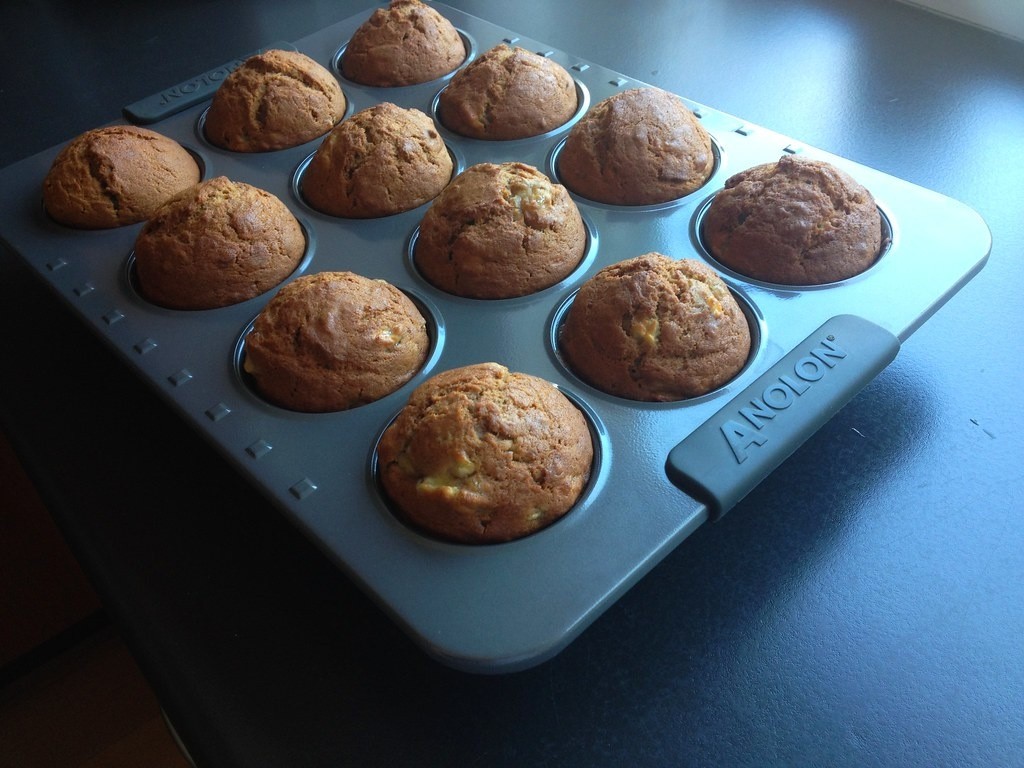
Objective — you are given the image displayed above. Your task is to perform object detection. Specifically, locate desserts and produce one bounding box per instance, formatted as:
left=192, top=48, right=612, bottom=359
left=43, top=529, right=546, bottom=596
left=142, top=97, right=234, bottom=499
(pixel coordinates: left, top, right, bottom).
left=43, top=0, right=880, bottom=541
